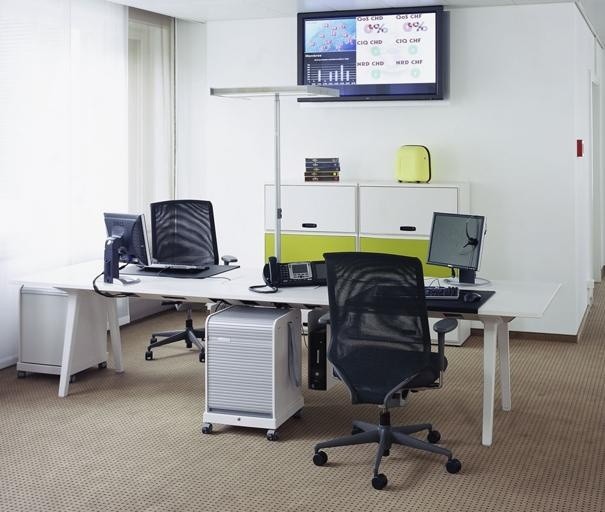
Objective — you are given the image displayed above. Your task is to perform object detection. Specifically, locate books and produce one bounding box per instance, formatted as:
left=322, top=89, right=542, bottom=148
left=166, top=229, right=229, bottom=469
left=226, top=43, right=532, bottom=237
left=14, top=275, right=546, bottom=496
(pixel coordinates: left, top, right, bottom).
left=305, top=158, right=340, bottom=182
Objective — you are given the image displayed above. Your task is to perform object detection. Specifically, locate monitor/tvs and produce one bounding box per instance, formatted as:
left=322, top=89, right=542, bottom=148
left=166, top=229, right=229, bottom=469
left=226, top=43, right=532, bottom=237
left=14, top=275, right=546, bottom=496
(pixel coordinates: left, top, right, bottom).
left=297, top=5, right=443, bottom=102
left=426, top=211, right=486, bottom=284
left=103, top=213, right=152, bottom=283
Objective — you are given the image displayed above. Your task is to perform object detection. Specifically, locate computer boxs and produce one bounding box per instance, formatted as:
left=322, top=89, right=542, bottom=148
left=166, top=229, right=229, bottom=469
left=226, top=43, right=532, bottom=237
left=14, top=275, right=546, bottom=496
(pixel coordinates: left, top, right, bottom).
left=307, top=309, right=341, bottom=391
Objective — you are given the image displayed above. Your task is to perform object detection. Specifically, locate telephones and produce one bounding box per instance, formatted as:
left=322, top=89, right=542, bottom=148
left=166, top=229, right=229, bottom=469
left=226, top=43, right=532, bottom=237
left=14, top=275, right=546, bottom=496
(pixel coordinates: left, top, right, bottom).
left=249, top=257, right=313, bottom=293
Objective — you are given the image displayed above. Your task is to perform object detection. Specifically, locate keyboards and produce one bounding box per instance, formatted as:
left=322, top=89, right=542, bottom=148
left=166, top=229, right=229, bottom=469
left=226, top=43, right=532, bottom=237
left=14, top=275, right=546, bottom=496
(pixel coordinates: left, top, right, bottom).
left=139, top=264, right=211, bottom=274
left=425, top=286, right=460, bottom=300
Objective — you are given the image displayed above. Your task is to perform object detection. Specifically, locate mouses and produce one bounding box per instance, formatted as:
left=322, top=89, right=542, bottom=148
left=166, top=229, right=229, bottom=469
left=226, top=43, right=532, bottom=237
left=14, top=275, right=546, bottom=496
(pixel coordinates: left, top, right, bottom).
left=462, top=292, right=482, bottom=304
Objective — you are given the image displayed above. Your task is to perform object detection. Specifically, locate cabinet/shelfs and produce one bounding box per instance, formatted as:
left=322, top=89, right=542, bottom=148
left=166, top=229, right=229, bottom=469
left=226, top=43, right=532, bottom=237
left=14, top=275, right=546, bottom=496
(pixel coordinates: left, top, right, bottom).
left=264, top=184, right=472, bottom=346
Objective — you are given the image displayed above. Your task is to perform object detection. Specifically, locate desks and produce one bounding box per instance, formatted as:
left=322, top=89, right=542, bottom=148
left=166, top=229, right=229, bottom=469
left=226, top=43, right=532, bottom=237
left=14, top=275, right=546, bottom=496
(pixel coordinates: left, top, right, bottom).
left=10, top=256, right=564, bottom=446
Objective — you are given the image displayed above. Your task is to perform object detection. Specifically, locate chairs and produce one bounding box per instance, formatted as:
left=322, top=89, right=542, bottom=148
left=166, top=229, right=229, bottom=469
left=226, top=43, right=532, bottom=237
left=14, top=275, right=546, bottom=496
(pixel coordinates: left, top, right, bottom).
left=313, top=252, right=461, bottom=490
left=144, top=199, right=238, bottom=363
